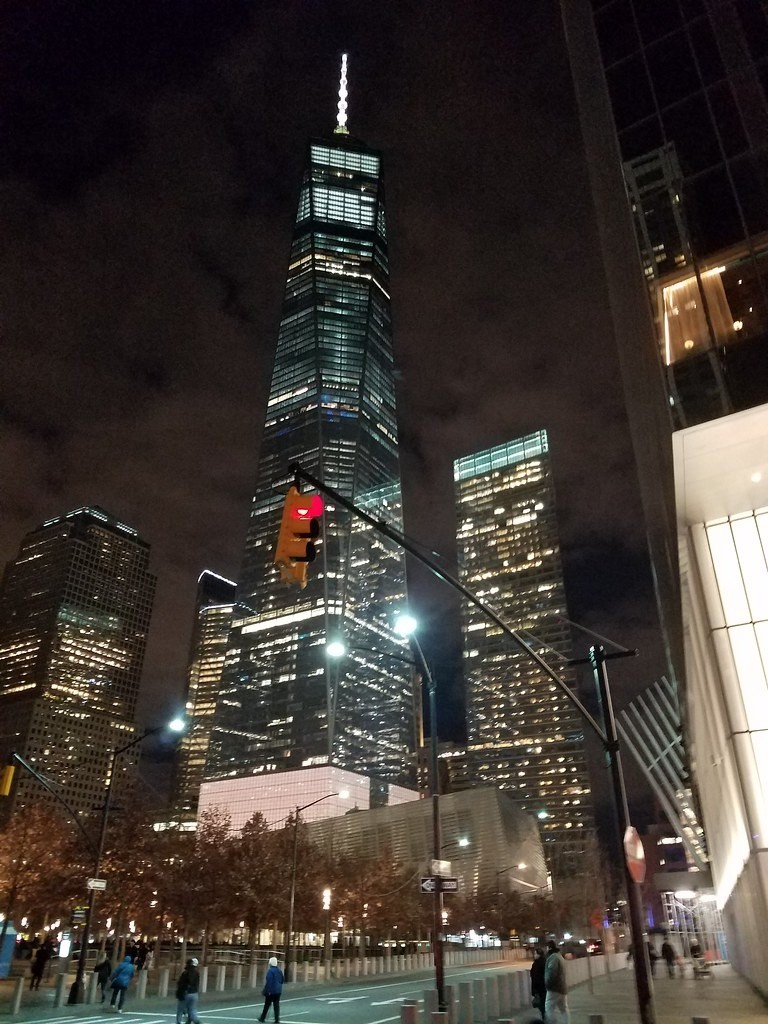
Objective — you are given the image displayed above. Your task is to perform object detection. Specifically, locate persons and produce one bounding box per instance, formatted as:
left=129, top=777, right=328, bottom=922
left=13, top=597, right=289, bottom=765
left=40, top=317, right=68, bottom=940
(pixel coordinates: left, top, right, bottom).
left=94, top=956, right=111, bottom=1004
left=44, top=936, right=56, bottom=956
left=140, top=943, right=155, bottom=969
left=176, top=958, right=199, bottom=1024
left=91, top=937, right=124, bottom=959
left=626, top=937, right=704, bottom=980
left=30, top=944, right=48, bottom=991
left=125, top=938, right=138, bottom=963
left=109, top=955, right=134, bottom=1014
left=525, top=938, right=572, bottom=1023
left=24, top=934, right=42, bottom=960
left=257, top=956, right=284, bottom=1024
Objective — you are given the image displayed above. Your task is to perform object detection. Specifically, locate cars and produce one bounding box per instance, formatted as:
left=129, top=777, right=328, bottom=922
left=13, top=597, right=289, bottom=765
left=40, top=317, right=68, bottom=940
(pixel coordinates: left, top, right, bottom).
left=585, top=939, right=604, bottom=956
left=558, top=941, right=589, bottom=960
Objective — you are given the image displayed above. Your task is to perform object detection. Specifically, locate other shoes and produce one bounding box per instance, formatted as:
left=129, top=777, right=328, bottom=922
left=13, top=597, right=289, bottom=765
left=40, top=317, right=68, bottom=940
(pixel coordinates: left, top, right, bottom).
left=118, top=1010, right=122, bottom=1013
left=257, top=1018, right=265, bottom=1023
left=110, top=1005, right=116, bottom=1009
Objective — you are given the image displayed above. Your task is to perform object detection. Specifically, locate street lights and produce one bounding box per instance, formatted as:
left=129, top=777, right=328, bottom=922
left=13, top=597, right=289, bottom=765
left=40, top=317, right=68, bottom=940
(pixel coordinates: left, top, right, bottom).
left=65, top=717, right=188, bottom=1007
left=396, top=611, right=448, bottom=1012
left=495, top=864, right=524, bottom=949
left=282, top=793, right=338, bottom=982
left=428, top=839, right=469, bottom=952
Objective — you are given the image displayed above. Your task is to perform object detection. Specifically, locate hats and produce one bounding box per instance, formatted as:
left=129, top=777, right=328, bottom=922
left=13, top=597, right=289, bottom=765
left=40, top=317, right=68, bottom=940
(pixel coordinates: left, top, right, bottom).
left=270, top=957, right=277, bottom=967
left=192, top=958, right=199, bottom=966
left=125, top=956, right=131, bottom=962
left=147, top=943, right=152, bottom=948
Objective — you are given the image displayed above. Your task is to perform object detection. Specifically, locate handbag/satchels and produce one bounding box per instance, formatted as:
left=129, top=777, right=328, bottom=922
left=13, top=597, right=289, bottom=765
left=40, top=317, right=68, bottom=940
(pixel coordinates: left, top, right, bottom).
left=110, top=977, right=119, bottom=989
